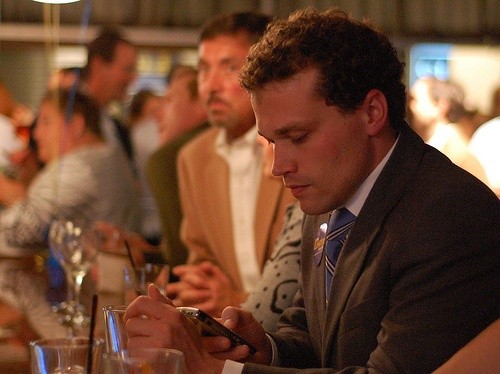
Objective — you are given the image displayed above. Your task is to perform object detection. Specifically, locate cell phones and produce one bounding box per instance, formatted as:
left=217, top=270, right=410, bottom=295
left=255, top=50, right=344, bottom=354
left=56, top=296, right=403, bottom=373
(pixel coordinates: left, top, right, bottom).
left=176, top=307, right=256, bottom=355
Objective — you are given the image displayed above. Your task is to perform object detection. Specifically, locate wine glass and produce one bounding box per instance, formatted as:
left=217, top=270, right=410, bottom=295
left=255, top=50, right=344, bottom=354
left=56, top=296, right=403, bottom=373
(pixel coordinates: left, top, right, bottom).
left=49, top=220, right=101, bottom=324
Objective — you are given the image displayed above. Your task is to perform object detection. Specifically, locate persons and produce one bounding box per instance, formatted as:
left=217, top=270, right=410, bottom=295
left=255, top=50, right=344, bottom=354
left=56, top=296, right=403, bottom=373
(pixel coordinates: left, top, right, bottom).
left=167, top=11, right=298, bottom=319
left=430, top=321, right=499, bottom=373
left=121, top=8, right=500, bottom=373
left=0, top=29, right=500, bottom=359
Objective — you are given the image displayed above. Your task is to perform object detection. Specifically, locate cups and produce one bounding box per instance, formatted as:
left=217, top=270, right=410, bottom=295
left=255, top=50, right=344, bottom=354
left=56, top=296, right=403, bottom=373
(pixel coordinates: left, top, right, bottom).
left=30, top=337, right=105, bottom=374
left=106, top=349, right=185, bottom=373
left=122, top=264, right=169, bottom=305
left=104, top=304, right=202, bottom=374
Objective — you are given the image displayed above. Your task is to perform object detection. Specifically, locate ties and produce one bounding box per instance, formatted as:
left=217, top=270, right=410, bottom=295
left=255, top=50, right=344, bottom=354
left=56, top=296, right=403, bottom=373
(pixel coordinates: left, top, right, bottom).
left=325, top=207, right=357, bottom=314
